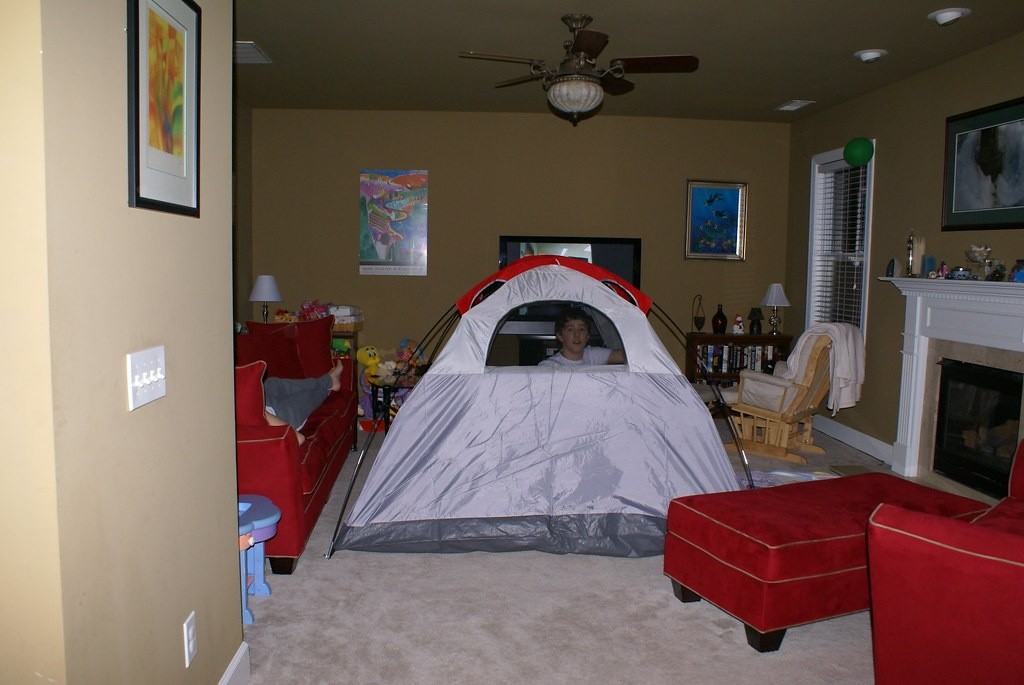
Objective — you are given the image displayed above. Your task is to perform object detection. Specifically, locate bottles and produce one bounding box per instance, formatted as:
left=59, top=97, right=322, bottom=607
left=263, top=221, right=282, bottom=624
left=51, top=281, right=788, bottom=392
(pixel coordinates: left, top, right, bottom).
left=712, top=304, right=726, bottom=334
left=1011, top=259, right=1024, bottom=283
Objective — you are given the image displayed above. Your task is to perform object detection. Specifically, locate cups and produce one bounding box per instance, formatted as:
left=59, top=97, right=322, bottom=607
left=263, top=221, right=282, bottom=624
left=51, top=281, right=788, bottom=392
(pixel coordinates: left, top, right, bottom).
left=921, top=255, right=936, bottom=278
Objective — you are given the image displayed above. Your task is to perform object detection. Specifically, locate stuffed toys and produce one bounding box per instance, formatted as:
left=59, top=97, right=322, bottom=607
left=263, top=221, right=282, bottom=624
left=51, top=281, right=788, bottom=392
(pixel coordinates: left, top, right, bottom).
left=355, top=339, right=421, bottom=386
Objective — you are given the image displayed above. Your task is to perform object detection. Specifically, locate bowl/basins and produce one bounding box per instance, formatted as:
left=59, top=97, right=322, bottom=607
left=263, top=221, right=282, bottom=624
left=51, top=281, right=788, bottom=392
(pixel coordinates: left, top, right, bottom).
left=965, top=250, right=991, bottom=262
left=951, top=270, right=971, bottom=280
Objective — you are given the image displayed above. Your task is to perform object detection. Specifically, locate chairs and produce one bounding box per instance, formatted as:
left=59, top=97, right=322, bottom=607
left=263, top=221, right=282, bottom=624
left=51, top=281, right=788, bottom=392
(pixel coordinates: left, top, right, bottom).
left=717, top=332, right=837, bottom=465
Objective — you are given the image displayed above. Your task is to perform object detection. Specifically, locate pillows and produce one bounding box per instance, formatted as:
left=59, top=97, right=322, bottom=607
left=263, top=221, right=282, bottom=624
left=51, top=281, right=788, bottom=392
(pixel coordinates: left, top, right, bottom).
left=234, top=360, right=270, bottom=429
left=263, top=322, right=309, bottom=381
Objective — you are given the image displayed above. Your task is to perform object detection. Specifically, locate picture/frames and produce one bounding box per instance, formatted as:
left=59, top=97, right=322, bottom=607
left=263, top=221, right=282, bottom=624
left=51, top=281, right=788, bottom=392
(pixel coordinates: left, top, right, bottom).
left=939, top=96, right=1023, bottom=232
left=685, top=179, right=749, bottom=263
left=126, top=0, right=201, bottom=219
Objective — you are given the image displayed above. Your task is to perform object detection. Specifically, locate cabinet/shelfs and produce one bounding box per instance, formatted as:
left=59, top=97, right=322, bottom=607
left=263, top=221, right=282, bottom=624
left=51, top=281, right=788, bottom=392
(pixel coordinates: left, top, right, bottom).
left=332, top=331, right=358, bottom=391
left=684, top=332, right=793, bottom=422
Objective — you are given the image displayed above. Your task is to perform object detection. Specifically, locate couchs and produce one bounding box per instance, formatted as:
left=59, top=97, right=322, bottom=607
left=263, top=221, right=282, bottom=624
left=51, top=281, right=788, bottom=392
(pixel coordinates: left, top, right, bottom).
left=234, top=317, right=358, bottom=576
left=867, top=437, right=1024, bottom=685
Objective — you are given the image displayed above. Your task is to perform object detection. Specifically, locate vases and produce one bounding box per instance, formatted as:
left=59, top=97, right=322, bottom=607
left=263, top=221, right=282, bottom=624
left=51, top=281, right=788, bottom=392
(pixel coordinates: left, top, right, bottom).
left=711, top=304, right=727, bottom=334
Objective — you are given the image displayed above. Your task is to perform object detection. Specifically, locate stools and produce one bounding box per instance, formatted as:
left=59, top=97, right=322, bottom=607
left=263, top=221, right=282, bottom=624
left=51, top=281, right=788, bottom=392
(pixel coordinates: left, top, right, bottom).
left=237, top=493, right=282, bottom=624
left=664, top=474, right=990, bottom=655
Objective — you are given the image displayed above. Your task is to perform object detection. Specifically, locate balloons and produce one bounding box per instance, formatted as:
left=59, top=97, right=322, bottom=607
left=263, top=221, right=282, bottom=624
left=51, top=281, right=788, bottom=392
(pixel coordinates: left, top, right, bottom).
left=843, top=137, right=874, bottom=168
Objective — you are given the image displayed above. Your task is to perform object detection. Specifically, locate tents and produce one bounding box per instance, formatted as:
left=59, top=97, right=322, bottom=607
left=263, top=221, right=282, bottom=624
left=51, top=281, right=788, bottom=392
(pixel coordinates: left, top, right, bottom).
left=325, top=255, right=754, bottom=558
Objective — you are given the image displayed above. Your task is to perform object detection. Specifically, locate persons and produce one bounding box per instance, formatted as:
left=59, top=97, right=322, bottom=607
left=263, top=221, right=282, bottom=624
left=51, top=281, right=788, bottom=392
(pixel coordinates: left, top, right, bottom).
left=539, top=310, right=627, bottom=366
left=263, top=360, right=343, bottom=445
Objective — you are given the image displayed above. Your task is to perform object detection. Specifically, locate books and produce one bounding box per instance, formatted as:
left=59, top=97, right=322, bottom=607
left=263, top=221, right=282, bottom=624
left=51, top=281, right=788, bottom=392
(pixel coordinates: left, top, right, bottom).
left=697, top=340, right=781, bottom=386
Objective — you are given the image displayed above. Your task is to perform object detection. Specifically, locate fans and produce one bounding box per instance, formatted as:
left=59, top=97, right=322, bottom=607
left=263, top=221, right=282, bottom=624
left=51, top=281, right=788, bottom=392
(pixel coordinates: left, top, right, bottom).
left=458, top=12, right=700, bottom=96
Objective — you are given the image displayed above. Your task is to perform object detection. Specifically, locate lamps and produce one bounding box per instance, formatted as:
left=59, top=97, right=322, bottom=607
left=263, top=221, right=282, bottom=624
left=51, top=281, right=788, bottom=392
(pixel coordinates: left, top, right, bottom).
left=760, top=284, right=792, bottom=335
left=543, top=73, right=604, bottom=123
left=747, top=307, right=765, bottom=337
left=248, top=275, right=282, bottom=323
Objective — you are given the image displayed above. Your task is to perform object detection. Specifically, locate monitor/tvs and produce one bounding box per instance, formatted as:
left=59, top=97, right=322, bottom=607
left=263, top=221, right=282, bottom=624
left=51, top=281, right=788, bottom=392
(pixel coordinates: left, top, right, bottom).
left=499, top=236, right=641, bottom=335
